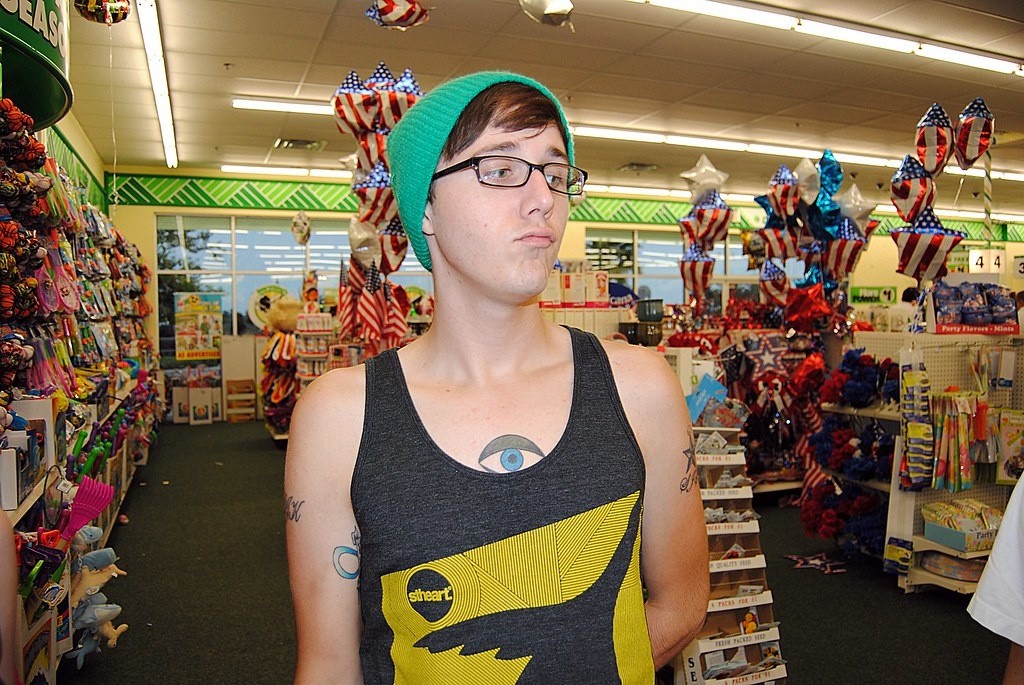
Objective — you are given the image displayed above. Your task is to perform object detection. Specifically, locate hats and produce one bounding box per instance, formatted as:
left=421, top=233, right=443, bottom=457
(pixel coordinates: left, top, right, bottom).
left=386, top=71, right=574, bottom=271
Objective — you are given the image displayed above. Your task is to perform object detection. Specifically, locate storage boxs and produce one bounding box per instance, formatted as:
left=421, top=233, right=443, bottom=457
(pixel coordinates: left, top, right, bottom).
left=925, top=522, right=999, bottom=552
left=1, top=395, right=56, bottom=512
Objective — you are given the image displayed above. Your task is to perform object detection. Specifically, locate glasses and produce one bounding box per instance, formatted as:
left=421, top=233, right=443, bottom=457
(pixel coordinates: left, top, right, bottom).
left=431, top=155, right=588, bottom=196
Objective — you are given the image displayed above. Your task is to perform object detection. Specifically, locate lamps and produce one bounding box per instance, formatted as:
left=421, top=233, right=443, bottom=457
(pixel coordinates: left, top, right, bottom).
left=630, top=1, right=1024, bottom=76
left=225, top=94, right=1024, bottom=182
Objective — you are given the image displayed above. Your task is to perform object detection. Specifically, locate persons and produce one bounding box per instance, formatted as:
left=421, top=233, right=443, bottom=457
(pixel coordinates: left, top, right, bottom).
left=283, top=69, right=711, bottom=685
left=200, top=316, right=210, bottom=335
left=0, top=508, right=22, bottom=685
left=966, top=470, right=1024, bottom=685
left=888, top=286, right=921, bottom=321
left=1016, top=290, right=1024, bottom=335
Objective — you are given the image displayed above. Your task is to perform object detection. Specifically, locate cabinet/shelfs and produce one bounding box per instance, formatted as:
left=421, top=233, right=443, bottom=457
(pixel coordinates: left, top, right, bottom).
left=1, top=361, right=156, bottom=685
left=294, top=329, right=333, bottom=400
left=226, top=394, right=255, bottom=413
left=671, top=426, right=787, bottom=684
left=817, top=331, right=1024, bottom=593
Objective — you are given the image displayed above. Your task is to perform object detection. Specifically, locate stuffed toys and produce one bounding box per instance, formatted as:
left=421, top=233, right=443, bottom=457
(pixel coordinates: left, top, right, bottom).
left=69, top=526, right=130, bottom=668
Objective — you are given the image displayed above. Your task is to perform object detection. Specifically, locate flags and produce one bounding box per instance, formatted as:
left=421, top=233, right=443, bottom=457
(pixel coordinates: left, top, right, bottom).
left=336, top=262, right=409, bottom=361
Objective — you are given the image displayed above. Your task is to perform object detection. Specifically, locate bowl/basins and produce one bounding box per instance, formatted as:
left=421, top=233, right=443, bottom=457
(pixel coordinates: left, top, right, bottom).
left=615, top=299, right=664, bottom=346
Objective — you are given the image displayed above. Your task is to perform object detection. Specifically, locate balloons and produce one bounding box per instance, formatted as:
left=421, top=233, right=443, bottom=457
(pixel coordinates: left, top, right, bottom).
left=680, top=98, right=995, bottom=307
left=330, top=62, right=426, bottom=277
left=365, top=0, right=430, bottom=32
